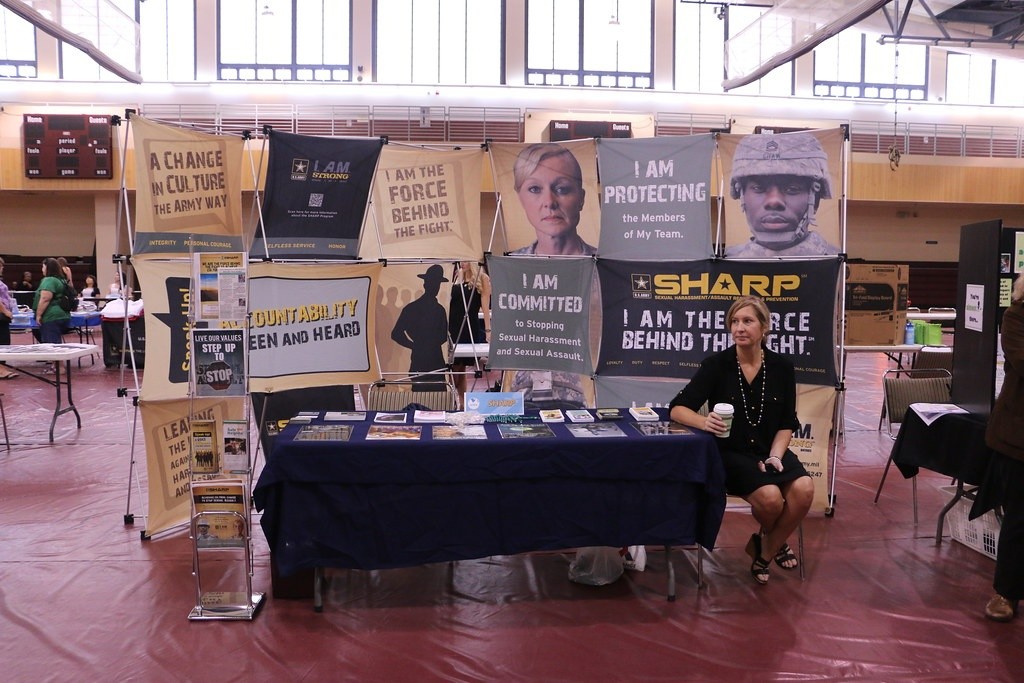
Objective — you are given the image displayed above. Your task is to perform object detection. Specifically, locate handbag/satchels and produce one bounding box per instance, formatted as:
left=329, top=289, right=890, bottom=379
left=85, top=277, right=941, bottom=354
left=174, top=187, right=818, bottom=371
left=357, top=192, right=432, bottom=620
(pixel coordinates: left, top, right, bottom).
left=567, top=546, right=626, bottom=587
left=616, top=546, right=649, bottom=572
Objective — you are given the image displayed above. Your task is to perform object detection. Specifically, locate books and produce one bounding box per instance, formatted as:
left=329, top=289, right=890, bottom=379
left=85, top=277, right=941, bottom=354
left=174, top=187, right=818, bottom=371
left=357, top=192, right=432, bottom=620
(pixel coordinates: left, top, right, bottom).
left=539, top=409, right=623, bottom=422
left=497, top=424, right=557, bottom=438
left=223, top=421, right=248, bottom=473
left=192, top=480, right=250, bottom=547
left=191, top=420, right=219, bottom=473
left=564, top=423, right=628, bottom=438
left=628, top=407, right=695, bottom=437
left=192, top=251, right=247, bottom=396
left=294, top=411, right=487, bottom=441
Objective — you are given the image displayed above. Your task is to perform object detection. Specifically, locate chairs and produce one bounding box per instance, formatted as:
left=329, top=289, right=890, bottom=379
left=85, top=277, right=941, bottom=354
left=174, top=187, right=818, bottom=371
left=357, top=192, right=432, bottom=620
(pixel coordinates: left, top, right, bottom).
left=367, top=380, right=455, bottom=411
left=698, top=402, right=805, bottom=588
left=873, top=368, right=957, bottom=523
left=910, top=345, right=954, bottom=378
left=906, top=307, right=955, bottom=334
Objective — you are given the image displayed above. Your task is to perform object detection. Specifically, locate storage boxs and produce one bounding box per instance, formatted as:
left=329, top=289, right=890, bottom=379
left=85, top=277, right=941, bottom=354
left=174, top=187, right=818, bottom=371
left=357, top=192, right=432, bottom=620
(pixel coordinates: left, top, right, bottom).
left=937, top=484, right=1004, bottom=561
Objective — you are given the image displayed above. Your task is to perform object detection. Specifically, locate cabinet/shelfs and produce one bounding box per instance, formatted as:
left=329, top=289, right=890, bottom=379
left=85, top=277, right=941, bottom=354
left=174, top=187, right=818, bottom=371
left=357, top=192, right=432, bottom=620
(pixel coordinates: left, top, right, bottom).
left=187, top=236, right=265, bottom=619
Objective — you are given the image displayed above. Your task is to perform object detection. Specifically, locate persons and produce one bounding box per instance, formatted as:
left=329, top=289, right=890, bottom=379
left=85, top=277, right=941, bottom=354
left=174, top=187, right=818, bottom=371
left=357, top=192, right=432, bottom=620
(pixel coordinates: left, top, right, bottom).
left=196, top=449, right=213, bottom=467
left=726, top=133, right=841, bottom=256
left=449, top=262, right=491, bottom=410
left=983, top=274, right=1024, bottom=622
left=669, top=295, right=815, bottom=585
left=225, top=439, right=245, bottom=455
left=507, top=143, right=598, bottom=256
left=196, top=519, right=217, bottom=540
left=0, top=256, right=133, bottom=379
left=228, top=519, right=245, bottom=540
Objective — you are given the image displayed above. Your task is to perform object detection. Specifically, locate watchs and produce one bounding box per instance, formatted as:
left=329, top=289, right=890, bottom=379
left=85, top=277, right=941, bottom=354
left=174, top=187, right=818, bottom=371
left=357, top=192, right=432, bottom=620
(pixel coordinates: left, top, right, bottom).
left=485, top=329, right=491, bottom=332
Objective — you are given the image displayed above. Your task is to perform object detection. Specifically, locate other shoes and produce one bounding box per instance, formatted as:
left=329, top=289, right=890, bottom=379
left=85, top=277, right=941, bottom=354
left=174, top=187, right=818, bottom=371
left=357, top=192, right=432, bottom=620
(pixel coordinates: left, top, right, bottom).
left=984, top=593, right=1017, bottom=621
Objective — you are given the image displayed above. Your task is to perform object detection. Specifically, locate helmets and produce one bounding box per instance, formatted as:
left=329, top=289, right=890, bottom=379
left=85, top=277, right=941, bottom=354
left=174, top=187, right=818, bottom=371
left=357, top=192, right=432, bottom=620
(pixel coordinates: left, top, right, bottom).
left=727, top=135, right=837, bottom=200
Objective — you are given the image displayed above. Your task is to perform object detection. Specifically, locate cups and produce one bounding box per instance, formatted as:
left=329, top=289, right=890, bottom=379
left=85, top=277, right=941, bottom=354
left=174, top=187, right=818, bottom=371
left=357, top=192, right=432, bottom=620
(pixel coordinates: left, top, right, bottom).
left=712, top=403, right=734, bottom=437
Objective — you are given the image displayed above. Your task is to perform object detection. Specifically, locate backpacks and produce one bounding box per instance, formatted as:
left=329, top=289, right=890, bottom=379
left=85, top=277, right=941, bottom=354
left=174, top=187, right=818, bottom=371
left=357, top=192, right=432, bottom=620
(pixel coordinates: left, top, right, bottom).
left=45, top=276, right=81, bottom=311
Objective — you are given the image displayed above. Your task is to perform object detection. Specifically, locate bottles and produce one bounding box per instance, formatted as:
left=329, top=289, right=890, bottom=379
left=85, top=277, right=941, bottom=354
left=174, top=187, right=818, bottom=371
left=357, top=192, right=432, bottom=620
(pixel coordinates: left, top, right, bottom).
left=906, top=322, right=914, bottom=345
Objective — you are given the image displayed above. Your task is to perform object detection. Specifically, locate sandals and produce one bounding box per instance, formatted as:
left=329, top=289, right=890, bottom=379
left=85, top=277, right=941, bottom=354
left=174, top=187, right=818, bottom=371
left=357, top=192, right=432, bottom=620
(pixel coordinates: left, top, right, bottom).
left=45, top=366, right=66, bottom=376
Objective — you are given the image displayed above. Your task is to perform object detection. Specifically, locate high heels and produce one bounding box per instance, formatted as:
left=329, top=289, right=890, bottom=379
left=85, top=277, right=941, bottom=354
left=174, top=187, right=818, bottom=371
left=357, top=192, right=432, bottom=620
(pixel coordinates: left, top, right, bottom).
left=744, top=533, right=771, bottom=584
left=760, top=527, right=799, bottom=570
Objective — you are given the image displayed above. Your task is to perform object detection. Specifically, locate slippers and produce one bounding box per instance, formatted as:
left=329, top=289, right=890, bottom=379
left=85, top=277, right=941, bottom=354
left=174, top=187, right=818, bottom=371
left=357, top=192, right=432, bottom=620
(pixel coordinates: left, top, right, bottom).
left=0, top=372, right=21, bottom=379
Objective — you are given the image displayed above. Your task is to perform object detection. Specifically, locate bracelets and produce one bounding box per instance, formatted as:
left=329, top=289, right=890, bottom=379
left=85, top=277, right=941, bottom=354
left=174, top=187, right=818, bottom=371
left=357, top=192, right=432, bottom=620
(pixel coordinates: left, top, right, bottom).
left=764, top=456, right=782, bottom=464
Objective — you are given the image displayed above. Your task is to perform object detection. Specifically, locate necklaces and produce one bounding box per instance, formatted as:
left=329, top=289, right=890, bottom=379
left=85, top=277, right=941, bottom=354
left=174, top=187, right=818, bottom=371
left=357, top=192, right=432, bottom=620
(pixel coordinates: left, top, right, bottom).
left=736, top=349, right=767, bottom=426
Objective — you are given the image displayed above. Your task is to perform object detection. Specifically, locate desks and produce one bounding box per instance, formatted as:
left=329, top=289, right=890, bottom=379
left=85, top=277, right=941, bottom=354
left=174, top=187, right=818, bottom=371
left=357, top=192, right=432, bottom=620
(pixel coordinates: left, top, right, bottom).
left=890, top=402, right=1008, bottom=544
left=101, top=309, right=145, bottom=369
left=9, top=311, right=102, bottom=369
left=0, top=343, right=99, bottom=442
left=7, top=291, right=37, bottom=311
left=836, top=343, right=924, bottom=430
left=887, top=312, right=956, bottom=365
left=74, top=297, right=131, bottom=310
left=252, top=343, right=726, bottom=613
